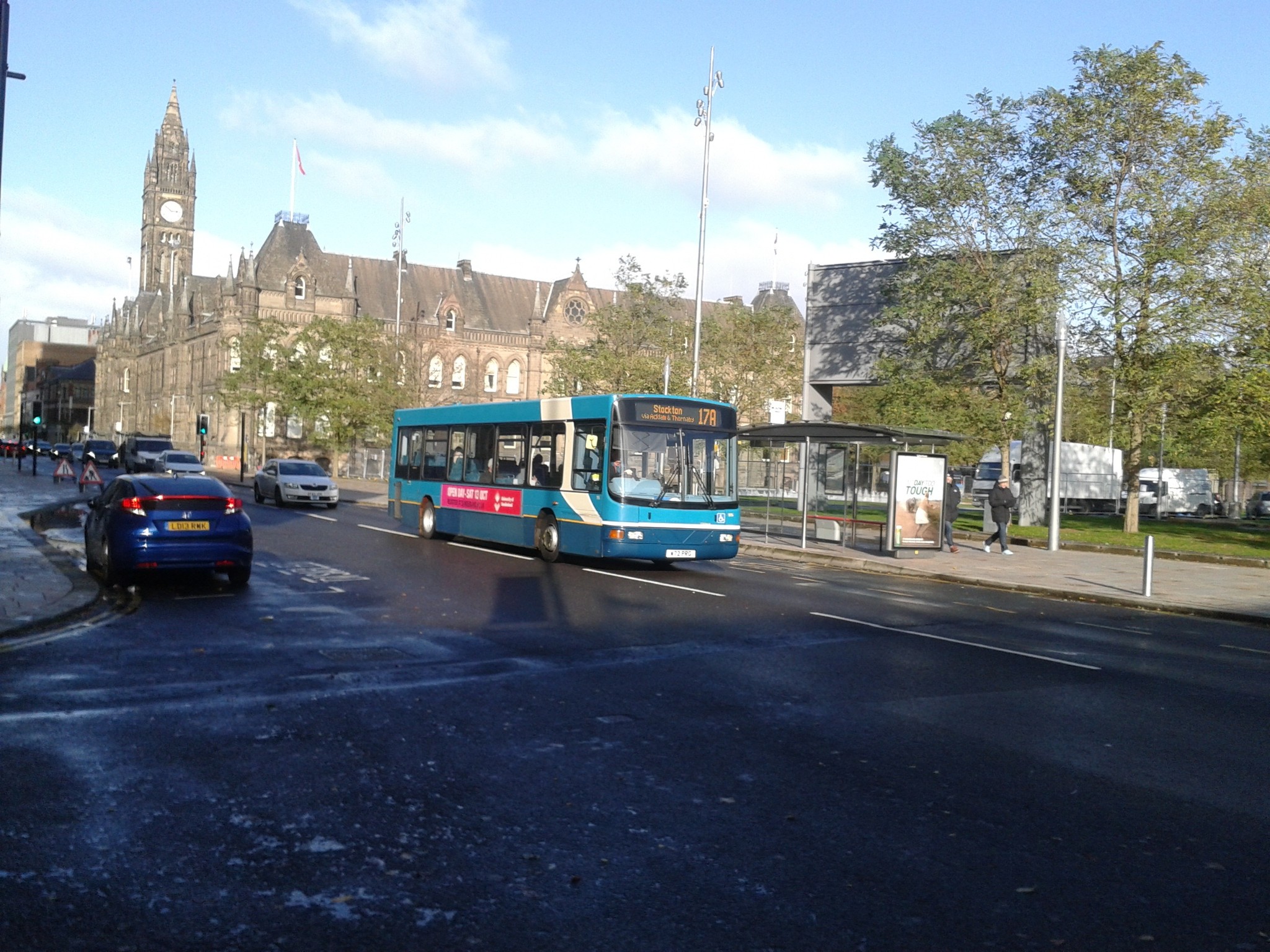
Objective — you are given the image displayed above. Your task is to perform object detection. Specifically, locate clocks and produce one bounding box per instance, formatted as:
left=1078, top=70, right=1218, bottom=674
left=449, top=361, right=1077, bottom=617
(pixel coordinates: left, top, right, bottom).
left=158, top=198, right=184, bottom=223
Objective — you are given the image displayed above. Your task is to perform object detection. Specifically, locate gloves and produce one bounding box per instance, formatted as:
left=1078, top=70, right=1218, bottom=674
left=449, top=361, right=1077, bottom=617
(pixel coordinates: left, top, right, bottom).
left=624, top=469, right=633, bottom=476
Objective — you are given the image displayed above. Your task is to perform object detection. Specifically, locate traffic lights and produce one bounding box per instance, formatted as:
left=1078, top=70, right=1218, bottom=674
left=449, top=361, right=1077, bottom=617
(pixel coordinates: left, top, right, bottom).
left=31, top=400, right=43, bottom=426
left=196, top=414, right=208, bottom=436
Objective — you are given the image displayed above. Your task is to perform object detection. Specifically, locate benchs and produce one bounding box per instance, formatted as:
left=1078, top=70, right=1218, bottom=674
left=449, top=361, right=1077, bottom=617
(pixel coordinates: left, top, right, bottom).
left=805, top=514, right=886, bottom=552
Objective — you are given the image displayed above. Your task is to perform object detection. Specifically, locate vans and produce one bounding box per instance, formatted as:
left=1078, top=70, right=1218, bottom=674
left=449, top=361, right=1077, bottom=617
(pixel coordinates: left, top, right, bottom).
left=1122, top=467, right=1213, bottom=518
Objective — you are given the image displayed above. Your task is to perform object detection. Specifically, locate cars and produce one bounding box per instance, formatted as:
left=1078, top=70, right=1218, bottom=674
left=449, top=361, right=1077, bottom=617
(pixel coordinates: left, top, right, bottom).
left=0, top=437, right=52, bottom=458
left=84, top=469, right=254, bottom=593
left=1245, top=490, right=1270, bottom=521
left=68, top=442, right=84, bottom=464
left=82, top=440, right=120, bottom=470
left=50, top=443, right=73, bottom=462
left=153, top=449, right=206, bottom=477
left=254, top=458, right=340, bottom=509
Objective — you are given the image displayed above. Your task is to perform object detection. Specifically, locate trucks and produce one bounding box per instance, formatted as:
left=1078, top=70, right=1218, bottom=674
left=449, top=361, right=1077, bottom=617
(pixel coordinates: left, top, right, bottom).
left=124, top=436, right=180, bottom=473
left=971, top=439, right=1124, bottom=517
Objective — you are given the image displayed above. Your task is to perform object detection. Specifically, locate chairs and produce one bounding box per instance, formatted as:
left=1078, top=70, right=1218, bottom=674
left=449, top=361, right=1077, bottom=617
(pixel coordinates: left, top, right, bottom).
left=400, top=452, right=526, bottom=486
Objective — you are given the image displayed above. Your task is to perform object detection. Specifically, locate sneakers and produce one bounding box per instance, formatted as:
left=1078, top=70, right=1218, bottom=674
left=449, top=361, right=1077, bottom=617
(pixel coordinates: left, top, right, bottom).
left=982, top=540, right=990, bottom=553
left=950, top=546, right=960, bottom=553
left=1002, top=550, right=1013, bottom=555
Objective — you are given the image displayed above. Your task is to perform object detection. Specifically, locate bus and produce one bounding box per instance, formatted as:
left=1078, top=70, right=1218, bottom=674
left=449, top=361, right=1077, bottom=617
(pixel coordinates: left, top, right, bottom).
left=388, top=394, right=741, bottom=567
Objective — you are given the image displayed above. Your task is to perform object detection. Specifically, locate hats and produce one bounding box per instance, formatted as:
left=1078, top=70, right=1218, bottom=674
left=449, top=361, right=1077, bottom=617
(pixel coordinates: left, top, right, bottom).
left=488, top=458, right=499, bottom=469
left=997, top=475, right=1009, bottom=483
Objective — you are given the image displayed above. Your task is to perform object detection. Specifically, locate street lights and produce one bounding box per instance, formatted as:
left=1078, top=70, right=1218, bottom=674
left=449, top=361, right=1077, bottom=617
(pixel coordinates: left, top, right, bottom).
left=692, top=46, right=726, bottom=393
left=1048, top=309, right=1068, bottom=552
left=1155, top=403, right=1167, bottom=519
left=391, top=195, right=411, bottom=362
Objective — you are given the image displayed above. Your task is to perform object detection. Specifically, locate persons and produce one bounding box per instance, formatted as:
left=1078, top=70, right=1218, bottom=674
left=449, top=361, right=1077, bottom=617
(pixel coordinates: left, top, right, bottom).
left=983, top=475, right=1015, bottom=555
left=914, top=495, right=933, bottom=538
left=1213, top=493, right=1223, bottom=516
left=610, top=452, right=633, bottom=480
left=118, top=441, right=126, bottom=463
left=479, top=458, right=499, bottom=484
left=532, top=454, right=549, bottom=486
left=945, top=472, right=961, bottom=553
